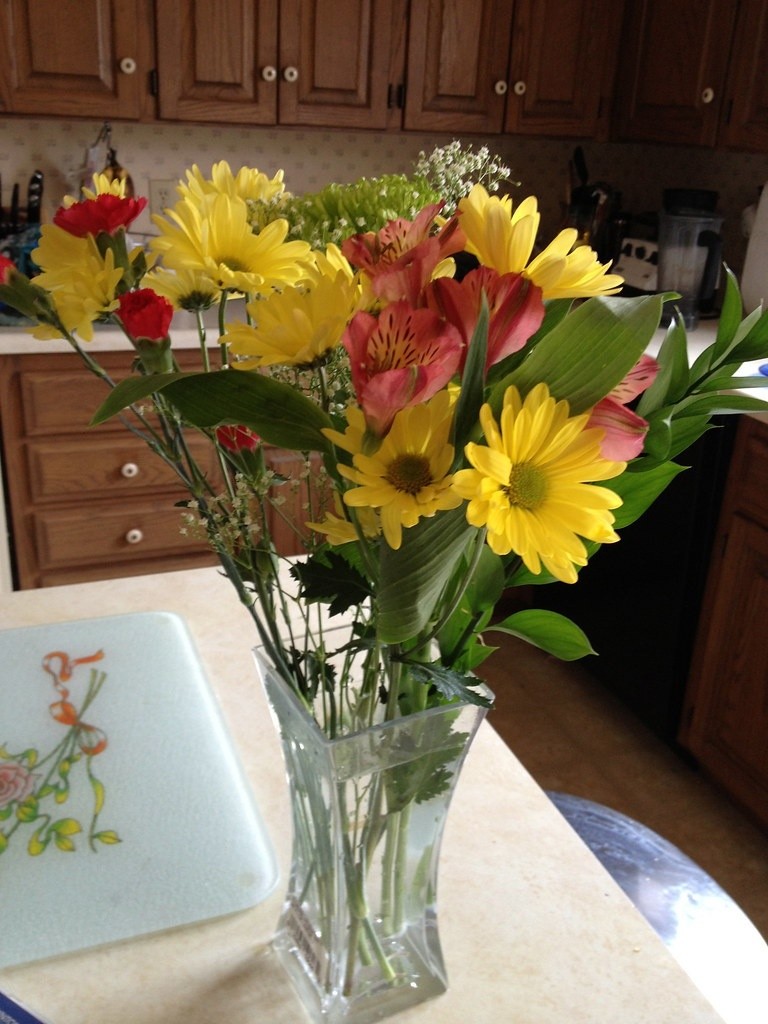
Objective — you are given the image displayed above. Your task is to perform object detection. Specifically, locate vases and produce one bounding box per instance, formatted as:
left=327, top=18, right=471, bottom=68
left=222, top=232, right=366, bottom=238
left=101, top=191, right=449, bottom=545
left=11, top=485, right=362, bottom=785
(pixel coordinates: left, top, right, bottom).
left=247, top=624, right=495, bottom=1024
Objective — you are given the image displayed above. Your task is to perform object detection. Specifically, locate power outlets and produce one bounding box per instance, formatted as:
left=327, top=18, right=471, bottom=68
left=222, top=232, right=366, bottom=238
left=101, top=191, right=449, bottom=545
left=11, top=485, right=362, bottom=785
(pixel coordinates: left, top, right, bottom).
left=148, top=179, right=176, bottom=224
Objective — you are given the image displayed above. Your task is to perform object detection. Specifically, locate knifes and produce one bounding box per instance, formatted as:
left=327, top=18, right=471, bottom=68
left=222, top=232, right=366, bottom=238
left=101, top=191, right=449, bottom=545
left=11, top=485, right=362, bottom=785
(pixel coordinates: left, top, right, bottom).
left=0, top=167, right=45, bottom=232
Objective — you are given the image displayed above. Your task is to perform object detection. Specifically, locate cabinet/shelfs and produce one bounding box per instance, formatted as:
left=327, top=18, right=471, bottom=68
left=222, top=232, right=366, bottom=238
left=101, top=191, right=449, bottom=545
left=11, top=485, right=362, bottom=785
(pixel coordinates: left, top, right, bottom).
left=0, top=350, right=768, bottom=823
left=0, top=0, right=768, bottom=155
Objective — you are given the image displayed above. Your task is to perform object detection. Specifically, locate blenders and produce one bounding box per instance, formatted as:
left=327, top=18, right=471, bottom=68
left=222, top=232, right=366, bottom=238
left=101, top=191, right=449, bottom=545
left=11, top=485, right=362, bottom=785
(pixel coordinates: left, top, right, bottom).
left=655, top=186, right=723, bottom=330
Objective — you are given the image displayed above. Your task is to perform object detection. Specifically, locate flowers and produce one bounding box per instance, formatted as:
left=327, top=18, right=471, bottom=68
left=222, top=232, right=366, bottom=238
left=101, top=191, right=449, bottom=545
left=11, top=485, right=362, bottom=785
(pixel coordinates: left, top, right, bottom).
left=0, top=138, right=768, bottom=992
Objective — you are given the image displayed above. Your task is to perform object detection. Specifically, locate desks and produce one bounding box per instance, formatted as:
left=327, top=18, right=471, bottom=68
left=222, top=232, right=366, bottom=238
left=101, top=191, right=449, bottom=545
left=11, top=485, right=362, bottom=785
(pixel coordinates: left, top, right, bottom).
left=0, top=552, right=719, bottom=1024
